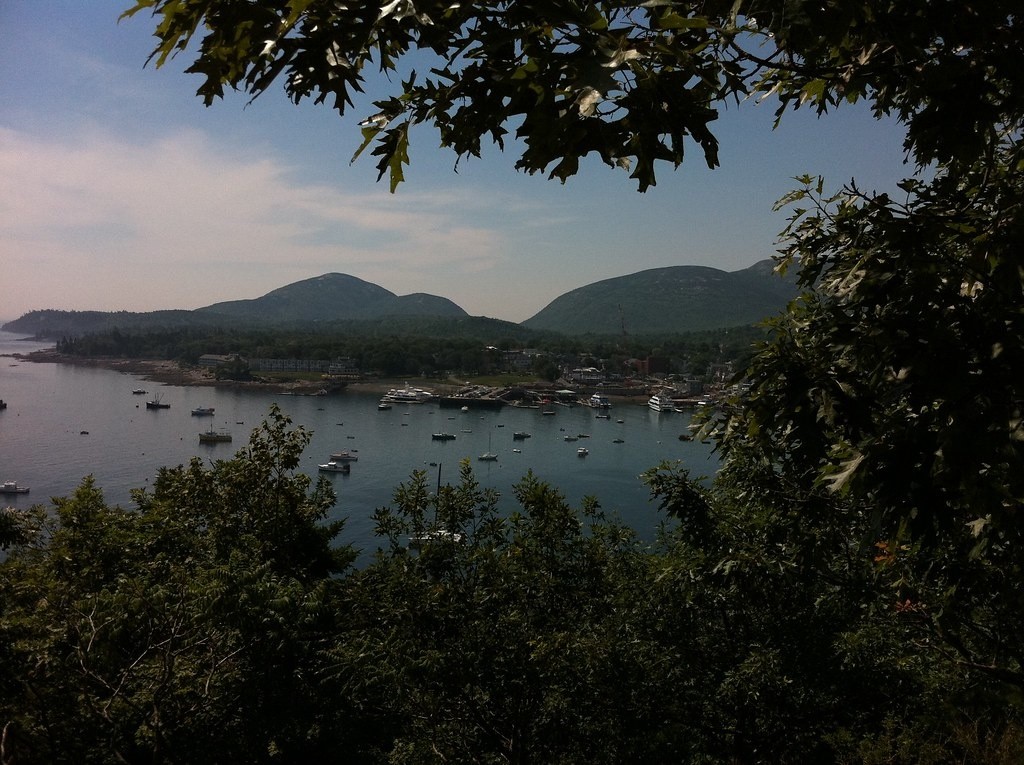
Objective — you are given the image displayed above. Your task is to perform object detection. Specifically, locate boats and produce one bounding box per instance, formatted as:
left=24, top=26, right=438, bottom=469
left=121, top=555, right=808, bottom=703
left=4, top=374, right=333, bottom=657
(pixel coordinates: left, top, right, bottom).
left=318, top=462, right=350, bottom=473
left=146, top=400, right=170, bottom=409
left=477, top=452, right=497, bottom=460
left=576, top=394, right=616, bottom=409
left=577, top=447, right=589, bottom=455
left=199, top=431, right=232, bottom=443
left=132, top=389, right=150, bottom=394
left=564, top=434, right=579, bottom=441
left=331, top=451, right=359, bottom=462
left=192, top=407, right=215, bottom=416
left=647, top=393, right=676, bottom=413
left=427, top=409, right=507, bottom=434
left=432, top=433, right=458, bottom=440
left=378, top=403, right=392, bottom=410
left=0, top=479, right=30, bottom=493
left=234, top=406, right=414, bottom=442
left=542, top=408, right=630, bottom=443
left=513, top=430, right=531, bottom=439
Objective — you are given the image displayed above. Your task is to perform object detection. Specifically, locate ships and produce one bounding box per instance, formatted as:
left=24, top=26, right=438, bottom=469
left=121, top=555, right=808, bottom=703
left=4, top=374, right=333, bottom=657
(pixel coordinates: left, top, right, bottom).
left=379, top=384, right=432, bottom=404
